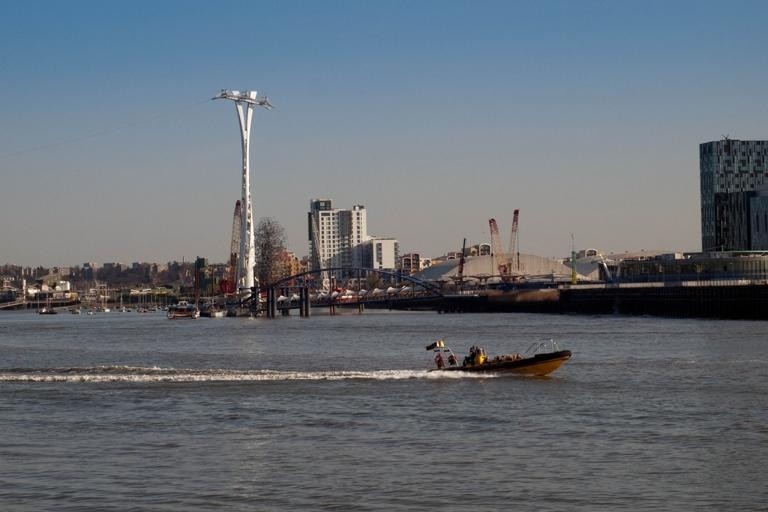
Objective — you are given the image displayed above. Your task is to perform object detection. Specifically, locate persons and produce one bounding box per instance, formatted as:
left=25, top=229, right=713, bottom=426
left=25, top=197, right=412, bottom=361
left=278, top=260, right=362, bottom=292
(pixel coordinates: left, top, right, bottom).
left=463, top=345, right=489, bottom=367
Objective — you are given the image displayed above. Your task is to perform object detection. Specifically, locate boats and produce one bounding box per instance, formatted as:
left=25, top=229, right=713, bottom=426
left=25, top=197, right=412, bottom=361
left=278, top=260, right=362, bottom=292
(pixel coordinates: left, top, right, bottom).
left=420, top=337, right=572, bottom=379
left=35, top=302, right=203, bottom=321
